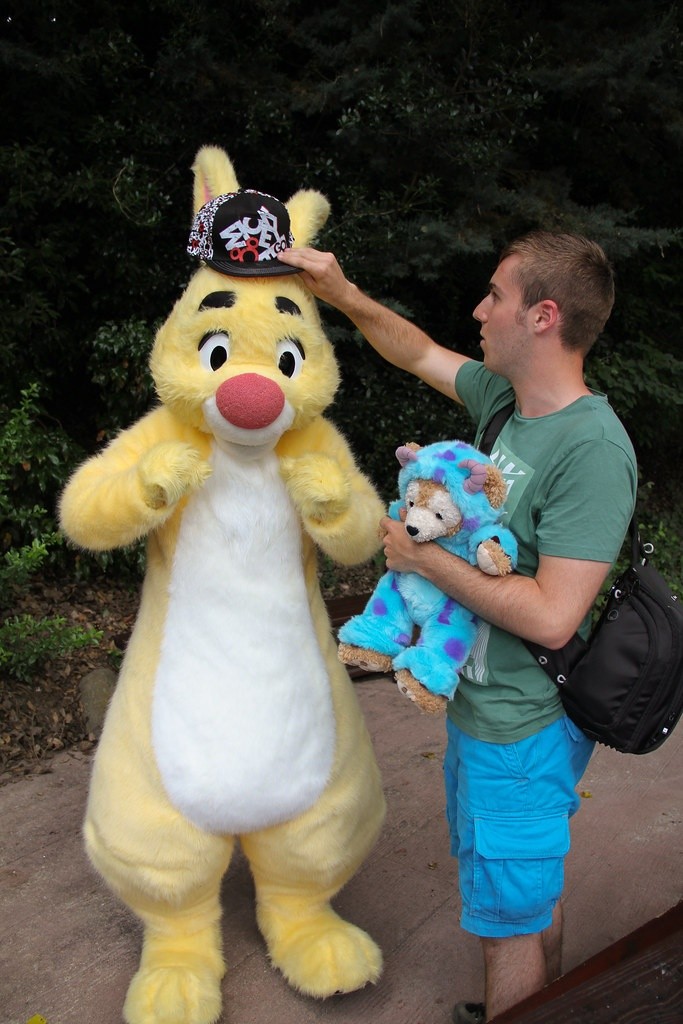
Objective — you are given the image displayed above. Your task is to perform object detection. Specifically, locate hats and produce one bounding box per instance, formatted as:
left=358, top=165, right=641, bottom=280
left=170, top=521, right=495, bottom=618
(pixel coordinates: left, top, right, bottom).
left=185, top=188, right=305, bottom=278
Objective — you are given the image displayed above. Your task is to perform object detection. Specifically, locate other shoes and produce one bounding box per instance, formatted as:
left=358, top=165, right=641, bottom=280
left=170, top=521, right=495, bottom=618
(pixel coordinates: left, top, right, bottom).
left=453, top=1000, right=485, bottom=1024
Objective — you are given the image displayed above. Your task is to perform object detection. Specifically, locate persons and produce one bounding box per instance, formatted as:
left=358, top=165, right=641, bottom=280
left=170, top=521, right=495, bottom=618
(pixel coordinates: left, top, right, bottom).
left=279, top=231, right=637, bottom=1024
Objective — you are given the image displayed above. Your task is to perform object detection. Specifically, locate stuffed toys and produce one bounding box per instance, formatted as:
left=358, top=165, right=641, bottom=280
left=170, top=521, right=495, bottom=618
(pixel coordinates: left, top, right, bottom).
left=337, top=440, right=518, bottom=714
left=59, top=143, right=392, bottom=1024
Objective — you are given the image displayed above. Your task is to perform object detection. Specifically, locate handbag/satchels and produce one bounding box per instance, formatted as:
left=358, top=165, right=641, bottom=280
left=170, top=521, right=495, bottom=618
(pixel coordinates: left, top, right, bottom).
left=559, top=562, right=683, bottom=755
